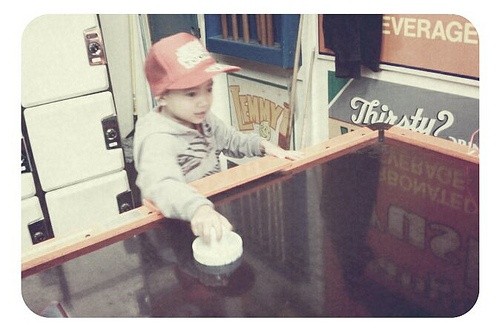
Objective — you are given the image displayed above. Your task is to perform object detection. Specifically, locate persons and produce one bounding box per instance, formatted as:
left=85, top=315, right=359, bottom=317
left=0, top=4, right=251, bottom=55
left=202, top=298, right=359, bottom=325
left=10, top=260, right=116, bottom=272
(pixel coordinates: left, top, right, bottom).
left=131, top=29, right=302, bottom=244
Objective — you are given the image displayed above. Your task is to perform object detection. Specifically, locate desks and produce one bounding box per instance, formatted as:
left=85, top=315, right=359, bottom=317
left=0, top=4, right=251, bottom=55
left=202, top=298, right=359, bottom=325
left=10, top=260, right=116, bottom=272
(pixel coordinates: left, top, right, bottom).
left=21, top=122, right=479, bottom=318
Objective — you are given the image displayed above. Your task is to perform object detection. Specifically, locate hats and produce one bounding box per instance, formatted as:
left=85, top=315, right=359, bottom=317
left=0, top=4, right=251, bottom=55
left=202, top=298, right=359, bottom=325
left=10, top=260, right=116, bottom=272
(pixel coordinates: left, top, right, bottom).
left=145, top=31, right=241, bottom=96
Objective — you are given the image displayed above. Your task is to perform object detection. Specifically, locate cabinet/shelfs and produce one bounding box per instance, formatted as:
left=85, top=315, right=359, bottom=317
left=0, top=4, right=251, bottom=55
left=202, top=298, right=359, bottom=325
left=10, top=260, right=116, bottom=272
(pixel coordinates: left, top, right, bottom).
left=20, top=14, right=135, bottom=250
